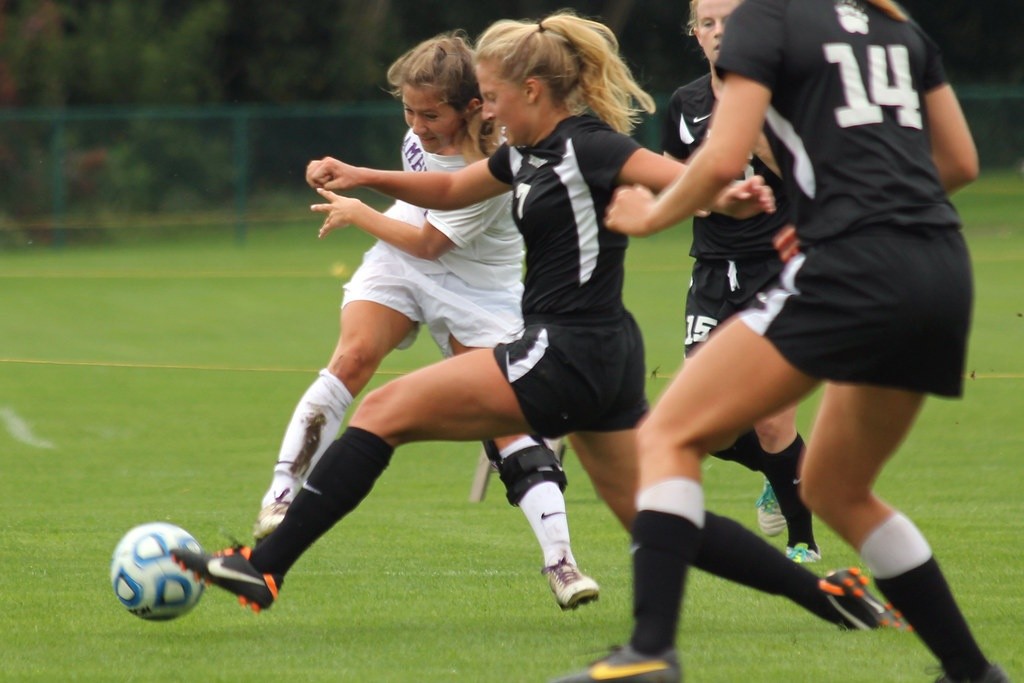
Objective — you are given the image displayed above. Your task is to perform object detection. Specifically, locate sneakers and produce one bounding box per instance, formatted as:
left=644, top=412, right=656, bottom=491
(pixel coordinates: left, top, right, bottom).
left=546, top=557, right=600, bottom=613
left=169, top=543, right=278, bottom=614
left=553, top=643, right=682, bottom=683
left=757, top=474, right=787, bottom=537
left=820, top=565, right=915, bottom=632
left=253, top=500, right=290, bottom=542
left=934, top=663, right=1009, bottom=683
left=783, top=542, right=822, bottom=565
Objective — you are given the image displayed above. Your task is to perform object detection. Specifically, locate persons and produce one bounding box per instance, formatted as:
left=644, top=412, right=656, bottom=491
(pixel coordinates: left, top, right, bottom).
left=660, top=0, right=823, bottom=563
left=168, top=12, right=912, bottom=632
left=550, top=0, right=1012, bottom=682
left=254, top=36, right=600, bottom=611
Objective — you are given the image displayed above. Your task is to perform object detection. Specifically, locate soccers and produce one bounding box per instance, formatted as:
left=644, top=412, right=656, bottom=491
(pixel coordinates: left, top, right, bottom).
left=111, top=521, right=205, bottom=624
